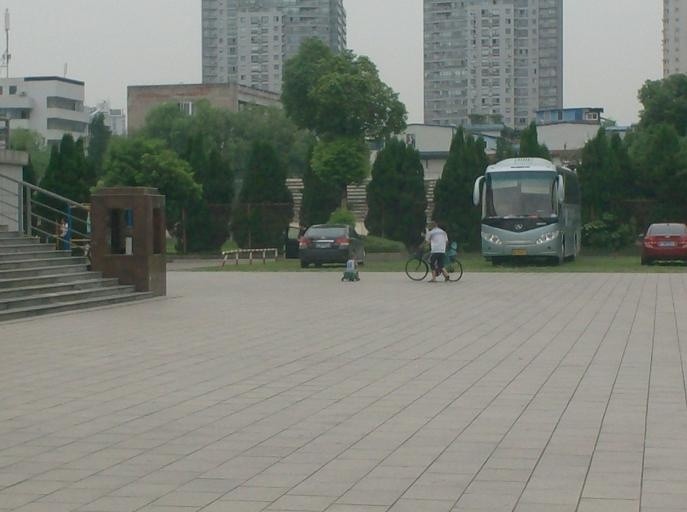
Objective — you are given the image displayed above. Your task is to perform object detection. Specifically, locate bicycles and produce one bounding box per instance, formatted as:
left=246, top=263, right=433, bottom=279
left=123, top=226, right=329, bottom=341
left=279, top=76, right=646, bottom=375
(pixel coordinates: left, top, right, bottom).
left=404, top=245, right=463, bottom=281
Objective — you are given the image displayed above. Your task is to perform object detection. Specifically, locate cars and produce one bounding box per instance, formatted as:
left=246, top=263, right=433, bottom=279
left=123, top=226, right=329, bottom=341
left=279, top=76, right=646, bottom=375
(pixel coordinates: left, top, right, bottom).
left=639, top=222, right=687, bottom=266
left=284, top=220, right=365, bottom=267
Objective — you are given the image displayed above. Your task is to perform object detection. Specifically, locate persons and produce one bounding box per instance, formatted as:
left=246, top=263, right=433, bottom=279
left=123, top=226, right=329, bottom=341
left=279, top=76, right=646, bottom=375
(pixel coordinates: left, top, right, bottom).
left=59, top=218, right=69, bottom=249
left=418, top=220, right=450, bottom=282
left=419, top=241, right=441, bottom=276
left=347, top=255, right=361, bottom=280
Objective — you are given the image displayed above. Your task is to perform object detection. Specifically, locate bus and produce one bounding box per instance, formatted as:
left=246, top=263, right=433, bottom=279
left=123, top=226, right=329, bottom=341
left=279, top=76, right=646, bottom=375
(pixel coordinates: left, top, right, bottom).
left=472, top=155, right=584, bottom=263
